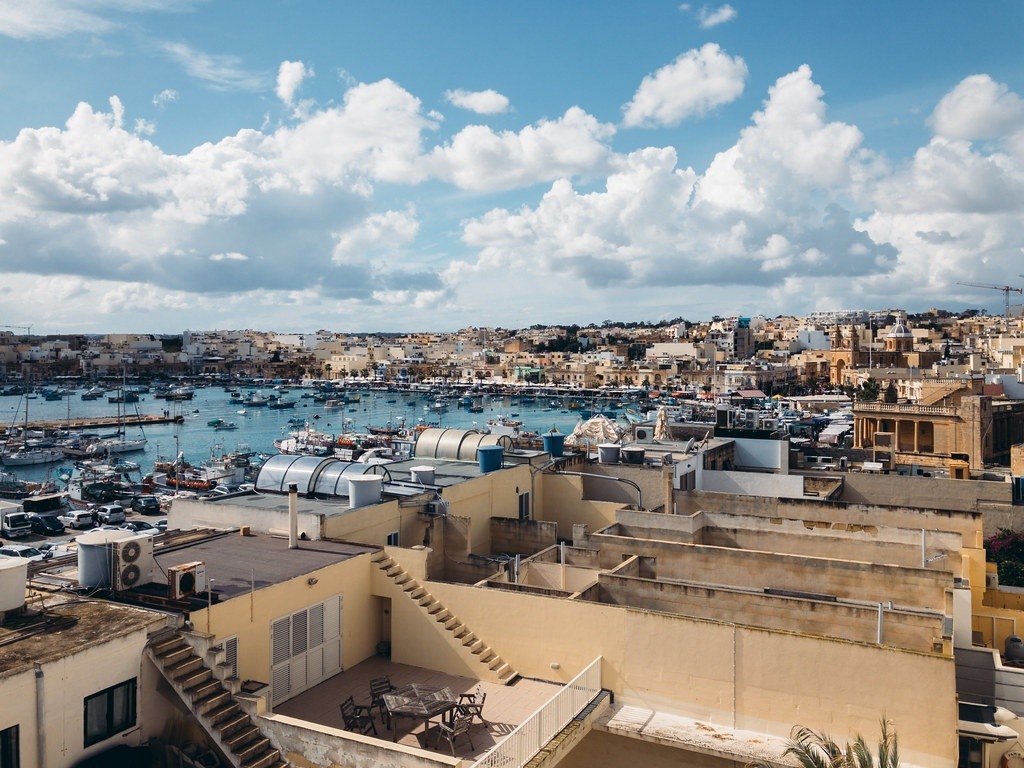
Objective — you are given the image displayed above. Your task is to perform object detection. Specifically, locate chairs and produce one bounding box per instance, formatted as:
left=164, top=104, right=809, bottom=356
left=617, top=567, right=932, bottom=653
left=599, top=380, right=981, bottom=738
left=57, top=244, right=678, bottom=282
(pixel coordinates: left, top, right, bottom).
left=435, top=710, right=475, bottom=758
left=340, top=696, right=378, bottom=737
left=370, top=678, right=399, bottom=724
left=453, top=684, right=490, bottom=729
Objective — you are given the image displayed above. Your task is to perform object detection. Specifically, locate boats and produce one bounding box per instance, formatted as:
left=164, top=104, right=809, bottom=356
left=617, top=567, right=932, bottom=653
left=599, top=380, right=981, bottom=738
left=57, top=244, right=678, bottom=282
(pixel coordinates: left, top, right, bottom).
left=0, top=372, right=677, bottom=560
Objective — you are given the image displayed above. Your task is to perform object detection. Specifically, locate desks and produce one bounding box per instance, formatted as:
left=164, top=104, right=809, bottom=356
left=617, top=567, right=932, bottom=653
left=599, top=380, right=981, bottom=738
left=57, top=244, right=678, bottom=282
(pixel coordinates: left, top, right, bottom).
left=383, top=682, right=457, bottom=743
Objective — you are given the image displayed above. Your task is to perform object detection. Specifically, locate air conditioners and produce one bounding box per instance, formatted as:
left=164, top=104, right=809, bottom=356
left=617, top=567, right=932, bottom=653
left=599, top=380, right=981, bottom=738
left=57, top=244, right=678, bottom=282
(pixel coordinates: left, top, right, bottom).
left=169, top=561, right=206, bottom=600
